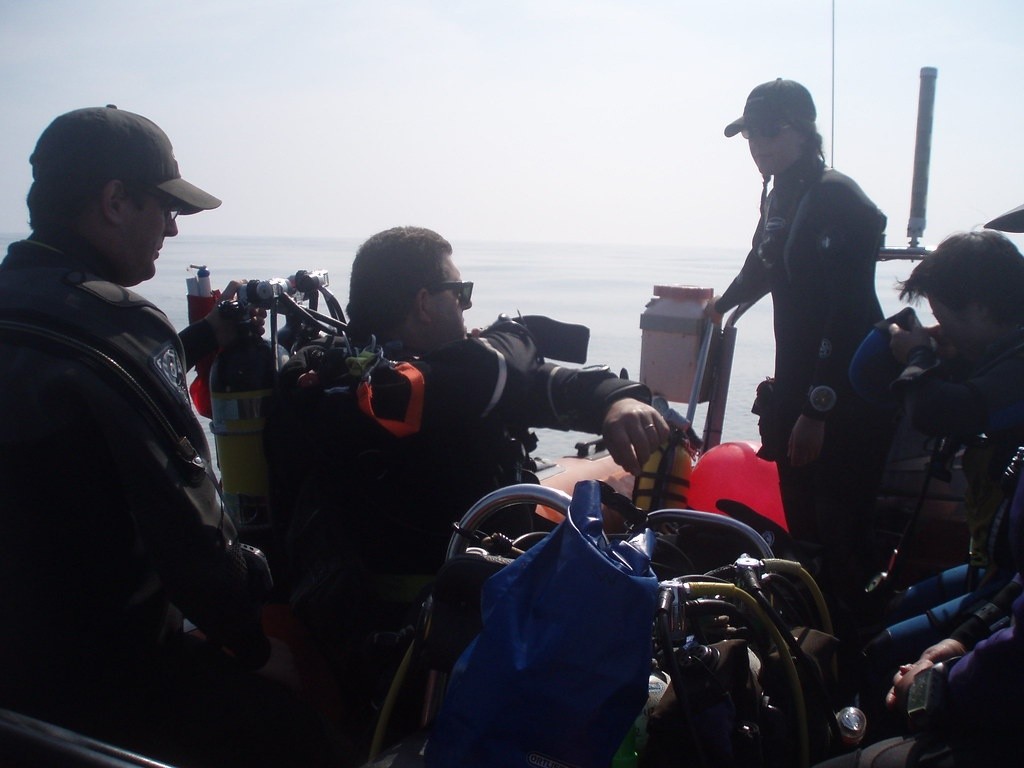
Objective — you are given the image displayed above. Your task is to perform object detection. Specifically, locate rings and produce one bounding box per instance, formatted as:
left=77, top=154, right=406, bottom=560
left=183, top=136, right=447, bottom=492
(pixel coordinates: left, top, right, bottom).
left=645, top=424, right=654, bottom=429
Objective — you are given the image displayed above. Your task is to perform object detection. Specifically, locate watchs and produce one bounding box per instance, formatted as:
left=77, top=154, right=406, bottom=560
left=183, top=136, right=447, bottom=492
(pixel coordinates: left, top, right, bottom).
left=905, top=656, right=963, bottom=732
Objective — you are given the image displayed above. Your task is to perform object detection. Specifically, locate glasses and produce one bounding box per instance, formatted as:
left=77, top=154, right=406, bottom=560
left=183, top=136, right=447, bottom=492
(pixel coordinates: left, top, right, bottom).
left=429, top=282, right=473, bottom=311
left=132, top=185, right=181, bottom=222
left=742, top=121, right=794, bottom=139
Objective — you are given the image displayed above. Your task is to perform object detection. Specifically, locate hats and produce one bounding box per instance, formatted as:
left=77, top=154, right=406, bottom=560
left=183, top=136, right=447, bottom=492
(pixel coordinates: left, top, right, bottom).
left=725, top=78, right=817, bottom=137
left=30, top=105, right=221, bottom=216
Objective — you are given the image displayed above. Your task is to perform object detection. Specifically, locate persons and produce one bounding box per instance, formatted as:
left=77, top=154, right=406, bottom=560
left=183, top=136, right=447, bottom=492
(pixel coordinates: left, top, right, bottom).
left=277, top=226, right=671, bottom=576
left=702, top=79, right=888, bottom=543
left=0, top=104, right=339, bottom=768
left=819, top=237, right=1024, bottom=768
